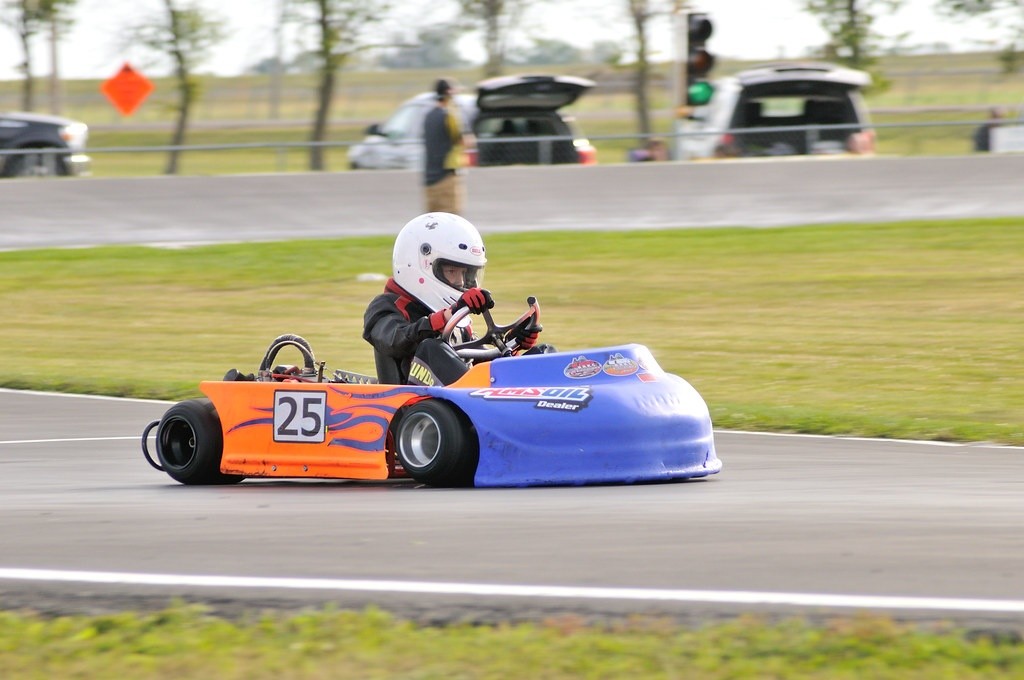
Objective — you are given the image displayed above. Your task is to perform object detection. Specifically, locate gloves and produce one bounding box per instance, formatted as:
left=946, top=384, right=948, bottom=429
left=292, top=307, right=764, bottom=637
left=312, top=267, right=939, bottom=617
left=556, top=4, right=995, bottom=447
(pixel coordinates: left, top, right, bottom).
left=508, top=323, right=543, bottom=350
left=451, top=287, right=494, bottom=315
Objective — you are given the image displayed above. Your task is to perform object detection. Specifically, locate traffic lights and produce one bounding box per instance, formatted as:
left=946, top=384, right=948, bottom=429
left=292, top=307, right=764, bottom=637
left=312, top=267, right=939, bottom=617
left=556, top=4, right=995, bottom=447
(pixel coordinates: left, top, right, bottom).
left=689, top=11, right=713, bottom=111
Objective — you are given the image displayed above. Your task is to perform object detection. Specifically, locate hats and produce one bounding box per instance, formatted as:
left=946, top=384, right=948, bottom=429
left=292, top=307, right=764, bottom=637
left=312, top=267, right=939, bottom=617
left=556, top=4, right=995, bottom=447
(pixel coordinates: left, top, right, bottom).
left=434, top=76, right=465, bottom=95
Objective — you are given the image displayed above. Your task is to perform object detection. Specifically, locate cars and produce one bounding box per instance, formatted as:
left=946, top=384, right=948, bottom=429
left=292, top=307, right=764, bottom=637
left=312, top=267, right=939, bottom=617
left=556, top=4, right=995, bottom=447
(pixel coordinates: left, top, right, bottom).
left=672, top=62, right=872, bottom=160
left=0, top=108, right=92, bottom=175
left=345, top=72, right=598, bottom=172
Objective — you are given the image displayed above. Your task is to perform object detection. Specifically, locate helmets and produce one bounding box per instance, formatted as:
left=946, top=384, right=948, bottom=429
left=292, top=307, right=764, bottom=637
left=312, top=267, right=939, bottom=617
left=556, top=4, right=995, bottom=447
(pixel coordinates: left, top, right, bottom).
left=393, top=212, right=487, bottom=328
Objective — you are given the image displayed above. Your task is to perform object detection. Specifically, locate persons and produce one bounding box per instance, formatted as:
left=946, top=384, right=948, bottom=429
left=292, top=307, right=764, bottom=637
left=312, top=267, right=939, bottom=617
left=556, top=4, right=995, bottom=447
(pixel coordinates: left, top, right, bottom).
left=417, top=73, right=481, bottom=217
left=971, top=104, right=1008, bottom=154
left=357, top=208, right=559, bottom=394
left=641, top=137, right=669, bottom=162
